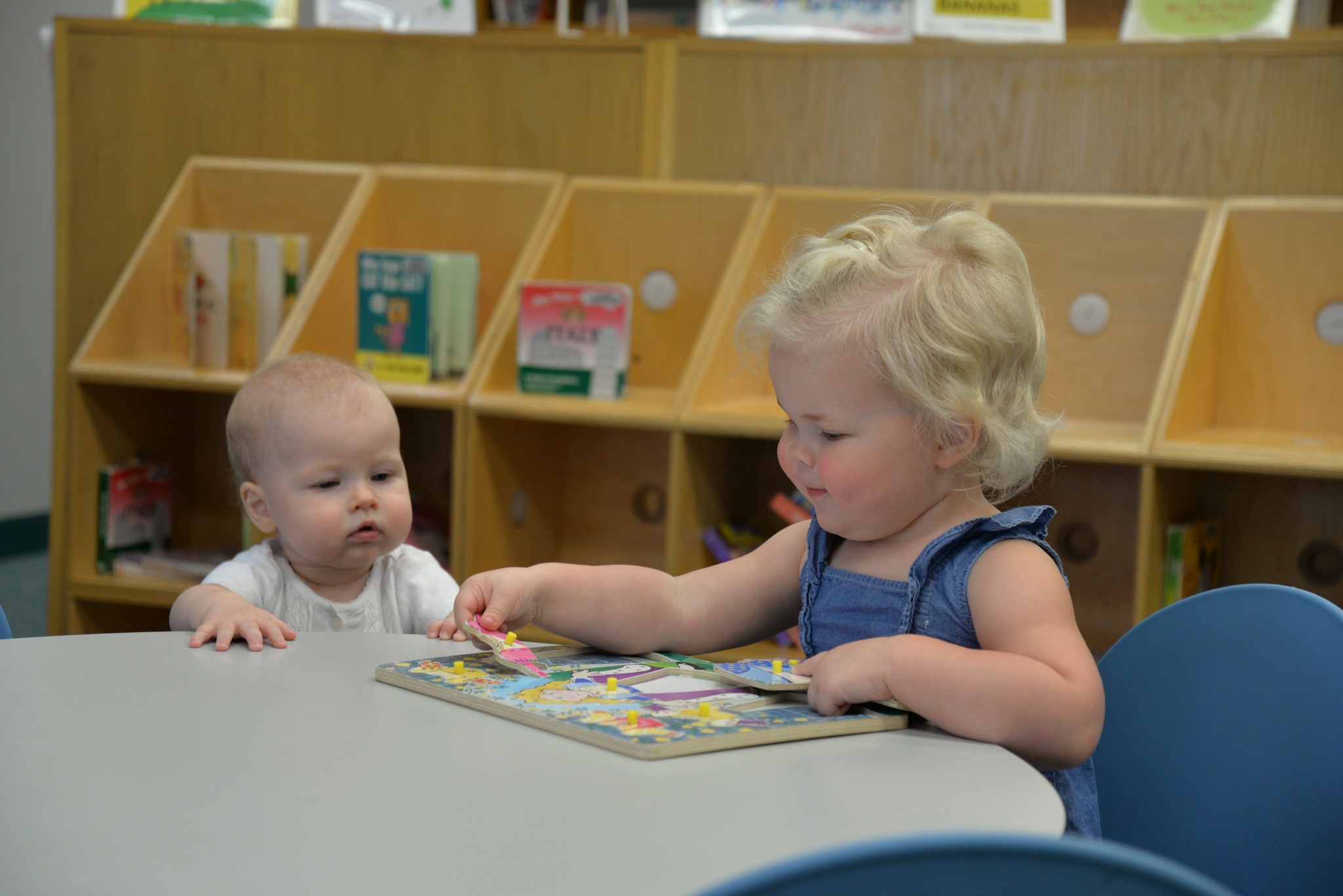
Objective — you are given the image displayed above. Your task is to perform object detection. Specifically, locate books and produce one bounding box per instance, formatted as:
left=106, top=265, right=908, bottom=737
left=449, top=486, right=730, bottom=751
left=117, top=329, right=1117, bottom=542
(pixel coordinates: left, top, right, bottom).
left=1165, top=516, right=1220, bottom=607
left=519, top=281, right=630, bottom=398
left=356, top=248, right=475, bottom=381
left=406, top=529, right=446, bottom=569
left=98, top=453, right=280, bottom=577
left=706, top=490, right=814, bottom=651
left=167, top=228, right=308, bottom=370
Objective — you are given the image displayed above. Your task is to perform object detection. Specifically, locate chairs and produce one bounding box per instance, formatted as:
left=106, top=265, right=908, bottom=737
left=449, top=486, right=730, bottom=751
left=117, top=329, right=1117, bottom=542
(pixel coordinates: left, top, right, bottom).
left=696, top=582, right=1343, bottom=896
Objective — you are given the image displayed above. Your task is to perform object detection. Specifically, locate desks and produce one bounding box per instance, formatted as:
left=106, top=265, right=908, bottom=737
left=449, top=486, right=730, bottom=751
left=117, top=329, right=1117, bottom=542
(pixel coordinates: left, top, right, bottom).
left=0, top=631, right=1067, bottom=896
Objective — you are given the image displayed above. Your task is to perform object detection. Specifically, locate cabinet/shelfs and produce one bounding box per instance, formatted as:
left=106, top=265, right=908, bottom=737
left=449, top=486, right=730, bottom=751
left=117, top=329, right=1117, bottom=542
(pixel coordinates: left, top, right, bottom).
left=61, top=154, right=1343, bottom=662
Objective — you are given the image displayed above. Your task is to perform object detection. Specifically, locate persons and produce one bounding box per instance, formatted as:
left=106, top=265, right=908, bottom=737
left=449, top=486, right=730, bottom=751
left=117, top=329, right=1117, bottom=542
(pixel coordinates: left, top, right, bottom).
left=170, top=357, right=470, bottom=652
left=453, top=204, right=1105, bottom=843
